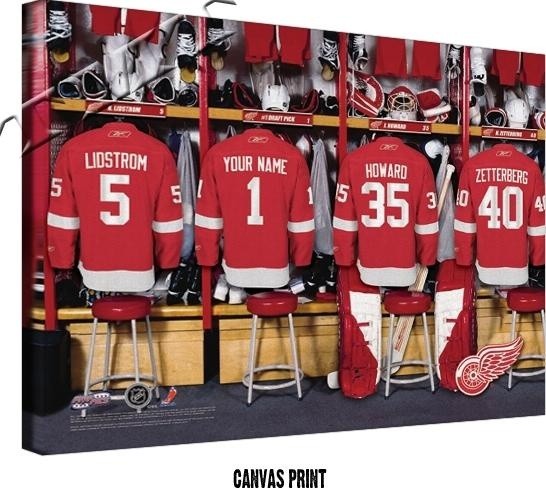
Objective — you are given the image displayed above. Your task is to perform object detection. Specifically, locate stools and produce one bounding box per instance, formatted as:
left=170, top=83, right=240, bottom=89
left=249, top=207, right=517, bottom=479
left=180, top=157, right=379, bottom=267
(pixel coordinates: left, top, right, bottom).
left=82, top=295, right=159, bottom=417
left=242, top=291, right=305, bottom=407
left=380, top=291, right=436, bottom=400
left=505, top=287, right=546, bottom=391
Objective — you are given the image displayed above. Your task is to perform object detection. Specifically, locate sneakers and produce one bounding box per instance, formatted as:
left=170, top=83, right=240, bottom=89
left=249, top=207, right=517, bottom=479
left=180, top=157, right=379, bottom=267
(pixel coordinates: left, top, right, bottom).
left=175, top=20, right=198, bottom=84
left=444, top=43, right=464, bottom=81
left=346, top=32, right=369, bottom=73
left=214, top=271, right=246, bottom=306
left=317, top=30, right=340, bottom=83
left=79, top=283, right=120, bottom=309
left=47, top=0, right=73, bottom=64
left=304, top=252, right=339, bottom=291
left=470, top=46, right=488, bottom=99
left=202, top=17, right=227, bottom=72
left=166, top=262, right=204, bottom=297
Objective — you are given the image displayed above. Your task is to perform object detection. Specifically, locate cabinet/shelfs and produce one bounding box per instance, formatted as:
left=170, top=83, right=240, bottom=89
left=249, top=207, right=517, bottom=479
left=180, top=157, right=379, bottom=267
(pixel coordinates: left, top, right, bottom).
left=26, top=299, right=546, bottom=393
left=22, top=1, right=545, bottom=334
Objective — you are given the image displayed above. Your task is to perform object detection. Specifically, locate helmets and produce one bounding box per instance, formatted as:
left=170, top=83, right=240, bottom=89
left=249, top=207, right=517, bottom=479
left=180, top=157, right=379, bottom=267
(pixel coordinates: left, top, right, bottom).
left=386, top=85, right=419, bottom=112
left=504, top=98, right=530, bottom=129
left=260, top=82, right=291, bottom=112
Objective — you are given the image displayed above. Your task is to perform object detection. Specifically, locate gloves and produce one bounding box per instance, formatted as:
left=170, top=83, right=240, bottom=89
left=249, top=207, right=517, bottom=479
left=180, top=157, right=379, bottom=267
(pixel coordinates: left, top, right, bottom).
left=483, top=106, right=508, bottom=129
left=417, top=86, right=453, bottom=123
